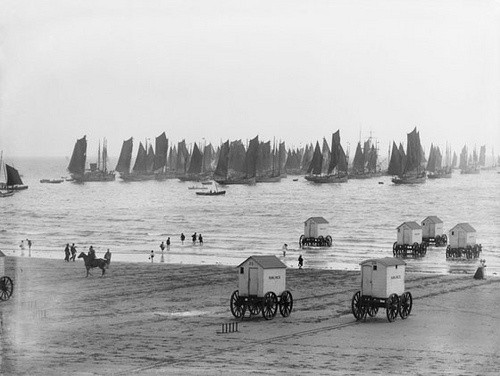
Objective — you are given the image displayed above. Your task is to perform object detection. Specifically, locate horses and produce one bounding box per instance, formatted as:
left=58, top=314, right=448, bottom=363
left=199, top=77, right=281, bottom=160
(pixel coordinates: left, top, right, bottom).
left=77, top=252, right=109, bottom=278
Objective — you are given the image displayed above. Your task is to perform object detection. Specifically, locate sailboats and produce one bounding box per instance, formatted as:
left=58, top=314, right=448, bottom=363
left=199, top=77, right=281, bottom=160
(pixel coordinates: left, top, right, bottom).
left=0, top=151, right=28, bottom=198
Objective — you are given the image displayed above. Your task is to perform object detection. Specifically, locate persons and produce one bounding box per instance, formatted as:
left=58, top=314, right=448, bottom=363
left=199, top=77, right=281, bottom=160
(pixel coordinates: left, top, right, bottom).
left=191, top=232, right=197, bottom=245
left=104, top=249, right=111, bottom=269
left=166, top=237, right=170, bottom=251
left=88, top=246, right=96, bottom=260
left=70, top=244, right=76, bottom=262
left=64, top=243, right=70, bottom=262
left=181, top=233, right=185, bottom=244
left=297, top=254, right=304, bottom=269
left=160, top=242, right=165, bottom=252
left=151, top=250, right=154, bottom=263
left=282, top=244, right=287, bottom=256
left=198, top=234, right=203, bottom=246
left=26, top=239, right=31, bottom=248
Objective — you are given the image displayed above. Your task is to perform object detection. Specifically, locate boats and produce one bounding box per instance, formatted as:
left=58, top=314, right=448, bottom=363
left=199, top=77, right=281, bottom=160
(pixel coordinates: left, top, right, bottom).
left=40, top=178, right=64, bottom=185
left=195, top=186, right=226, bottom=196
